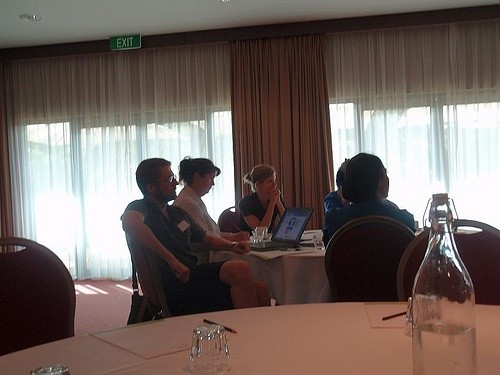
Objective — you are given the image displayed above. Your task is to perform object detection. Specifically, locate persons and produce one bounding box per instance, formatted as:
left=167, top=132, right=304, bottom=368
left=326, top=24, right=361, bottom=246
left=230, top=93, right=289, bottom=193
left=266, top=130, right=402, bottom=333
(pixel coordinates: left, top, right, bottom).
left=240, top=163, right=285, bottom=234
left=120, top=157, right=269, bottom=310
left=322, top=153, right=415, bottom=251
left=171, top=155, right=250, bottom=263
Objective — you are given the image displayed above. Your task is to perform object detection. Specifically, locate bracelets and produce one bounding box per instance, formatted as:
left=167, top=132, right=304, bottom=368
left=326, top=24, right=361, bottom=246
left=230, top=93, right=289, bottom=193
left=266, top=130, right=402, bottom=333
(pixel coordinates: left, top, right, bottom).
left=231, top=241, right=238, bottom=251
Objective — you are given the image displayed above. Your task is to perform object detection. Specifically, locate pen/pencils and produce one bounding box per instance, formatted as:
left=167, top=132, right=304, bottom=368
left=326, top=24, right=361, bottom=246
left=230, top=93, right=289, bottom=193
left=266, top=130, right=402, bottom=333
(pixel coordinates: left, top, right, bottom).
left=203, top=319, right=238, bottom=333
left=382, top=311, right=407, bottom=320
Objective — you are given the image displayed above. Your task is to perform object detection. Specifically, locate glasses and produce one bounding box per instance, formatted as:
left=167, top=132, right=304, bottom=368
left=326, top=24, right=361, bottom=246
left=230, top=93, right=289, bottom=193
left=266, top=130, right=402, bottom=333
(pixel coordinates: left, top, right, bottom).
left=160, top=175, right=177, bottom=182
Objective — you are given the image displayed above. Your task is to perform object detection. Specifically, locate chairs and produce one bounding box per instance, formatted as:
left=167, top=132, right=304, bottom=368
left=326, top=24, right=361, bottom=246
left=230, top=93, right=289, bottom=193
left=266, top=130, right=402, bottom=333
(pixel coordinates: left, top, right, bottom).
left=126, top=207, right=239, bottom=324
left=0, top=237, right=75, bottom=357
left=324, top=216, right=500, bottom=306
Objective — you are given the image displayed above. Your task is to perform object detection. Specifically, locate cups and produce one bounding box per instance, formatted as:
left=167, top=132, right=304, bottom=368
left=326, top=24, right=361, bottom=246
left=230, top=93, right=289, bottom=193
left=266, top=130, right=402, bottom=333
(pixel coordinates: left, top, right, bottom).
left=253, top=231, right=264, bottom=248
left=191, top=325, right=231, bottom=375
left=256, top=227, right=267, bottom=241
left=406, top=296, right=440, bottom=336
left=313, top=233, right=325, bottom=250
left=31, top=365, right=70, bottom=375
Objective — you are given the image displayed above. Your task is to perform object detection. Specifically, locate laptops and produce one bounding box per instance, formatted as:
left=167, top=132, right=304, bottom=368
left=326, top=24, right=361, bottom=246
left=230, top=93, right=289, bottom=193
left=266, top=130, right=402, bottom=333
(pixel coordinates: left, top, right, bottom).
left=250, top=208, right=315, bottom=252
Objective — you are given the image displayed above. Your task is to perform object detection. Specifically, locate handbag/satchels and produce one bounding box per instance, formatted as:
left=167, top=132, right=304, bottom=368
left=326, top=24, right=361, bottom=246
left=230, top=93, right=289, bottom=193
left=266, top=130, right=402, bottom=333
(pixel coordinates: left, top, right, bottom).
left=127, top=294, right=163, bottom=326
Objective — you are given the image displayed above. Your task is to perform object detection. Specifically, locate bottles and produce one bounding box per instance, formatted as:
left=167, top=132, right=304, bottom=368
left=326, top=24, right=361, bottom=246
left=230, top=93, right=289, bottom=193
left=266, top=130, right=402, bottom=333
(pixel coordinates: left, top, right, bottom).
left=411, top=193, right=476, bottom=375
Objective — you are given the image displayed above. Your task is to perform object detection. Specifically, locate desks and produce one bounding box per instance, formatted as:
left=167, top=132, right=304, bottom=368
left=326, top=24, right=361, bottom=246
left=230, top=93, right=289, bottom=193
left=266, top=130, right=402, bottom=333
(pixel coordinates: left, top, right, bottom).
left=0, top=302, right=500, bottom=375
left=210, top=231, right=331, bottom=306
